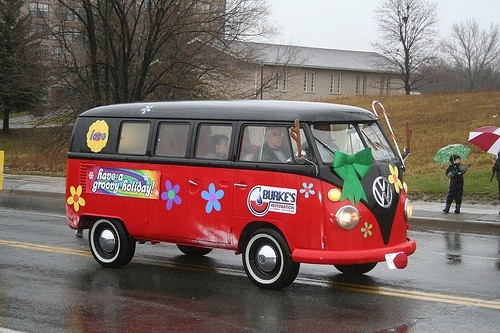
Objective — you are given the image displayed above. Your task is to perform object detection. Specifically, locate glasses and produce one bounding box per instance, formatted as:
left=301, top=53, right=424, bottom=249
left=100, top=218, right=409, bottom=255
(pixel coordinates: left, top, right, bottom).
left=269, top=131, right=284, bottom=137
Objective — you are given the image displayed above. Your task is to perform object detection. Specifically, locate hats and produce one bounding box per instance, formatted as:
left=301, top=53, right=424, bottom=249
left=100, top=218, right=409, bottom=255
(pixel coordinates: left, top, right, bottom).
left=450, top=155, right=460, bottom=163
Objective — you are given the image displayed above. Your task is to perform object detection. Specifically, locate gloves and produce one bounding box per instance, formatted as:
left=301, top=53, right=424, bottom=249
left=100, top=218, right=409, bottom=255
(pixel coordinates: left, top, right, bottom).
left=452, top=164, right=459, bottom=168
left=493, top=166, right=497, bottom=174
left=453, top=167, right=458, bottom=172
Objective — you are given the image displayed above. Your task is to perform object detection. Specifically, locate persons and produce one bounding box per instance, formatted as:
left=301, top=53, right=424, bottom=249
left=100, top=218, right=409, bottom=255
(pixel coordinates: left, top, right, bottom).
left=208, top=134, right=229, bottom=159
left=442, top=155, right=468, bottom=213
left=491, top=151, right=500, bottom=214
left=302, top=123, right=341, bottom=162
left=256, top=126, right=290, bottom=162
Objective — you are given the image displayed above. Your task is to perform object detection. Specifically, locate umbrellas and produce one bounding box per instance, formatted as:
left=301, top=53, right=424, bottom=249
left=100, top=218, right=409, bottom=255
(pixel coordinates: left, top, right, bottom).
left=433, top=145, right=471, bottom=173
left=468, top=126, right=500, bottom=155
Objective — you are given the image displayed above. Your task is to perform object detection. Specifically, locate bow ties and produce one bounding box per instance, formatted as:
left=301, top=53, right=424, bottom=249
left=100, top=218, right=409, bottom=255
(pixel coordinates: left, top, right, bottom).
left=271, top=148, right=280, bottom=151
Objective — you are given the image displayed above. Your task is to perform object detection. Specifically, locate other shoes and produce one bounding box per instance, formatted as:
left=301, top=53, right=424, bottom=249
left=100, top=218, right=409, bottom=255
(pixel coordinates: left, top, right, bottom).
left=455, top=209, right=460, bottom=213
left=443, top=208, right=449, bottom=213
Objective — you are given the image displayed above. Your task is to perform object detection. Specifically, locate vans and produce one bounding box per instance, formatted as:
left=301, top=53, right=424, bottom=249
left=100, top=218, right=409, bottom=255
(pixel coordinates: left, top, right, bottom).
left=64, top=98, right=417, bottom=291
